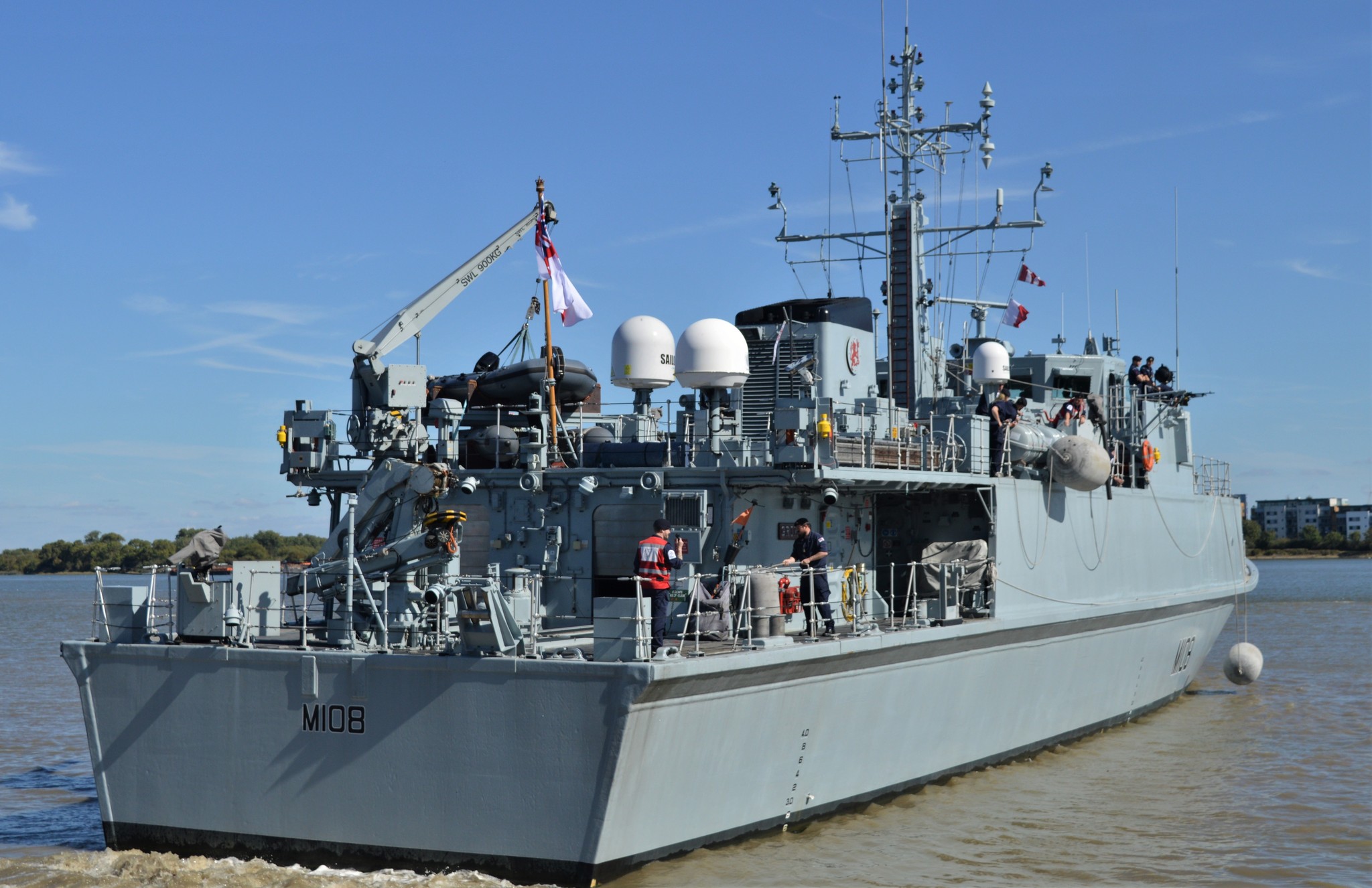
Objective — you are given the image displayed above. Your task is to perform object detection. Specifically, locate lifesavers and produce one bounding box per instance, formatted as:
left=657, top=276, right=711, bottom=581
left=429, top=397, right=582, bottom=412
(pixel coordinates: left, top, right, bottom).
left=1142, top=440, right=1154, bottom=472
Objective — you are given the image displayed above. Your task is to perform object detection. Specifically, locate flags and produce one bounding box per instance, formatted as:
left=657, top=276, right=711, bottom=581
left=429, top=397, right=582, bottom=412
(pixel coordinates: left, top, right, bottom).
left=1016, top=264, right=1045, bottom=287
left=535, top=192, right=593, bottom=327
left=1002, top=299, right=1029, bottom=328
left=731, top=505, right=753, bottom=527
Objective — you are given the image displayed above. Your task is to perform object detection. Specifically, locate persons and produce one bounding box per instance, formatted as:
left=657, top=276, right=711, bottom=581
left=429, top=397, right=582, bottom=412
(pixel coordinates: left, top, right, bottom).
left=782, top=518, right=835, bottom=636
left=634, top=519, right=684, bottom=656
left=1128, top=355, right=1155, bottom=409
left=1053, top=393, right=1086, bottom=429
left=976, top=386, right=1027, bottom=476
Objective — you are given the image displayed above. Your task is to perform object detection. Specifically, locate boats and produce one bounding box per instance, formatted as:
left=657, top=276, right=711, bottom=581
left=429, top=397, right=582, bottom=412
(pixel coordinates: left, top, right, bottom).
left=59, top=0, right=1259, bottom=888
left=427, top=357, right=598, bottom=405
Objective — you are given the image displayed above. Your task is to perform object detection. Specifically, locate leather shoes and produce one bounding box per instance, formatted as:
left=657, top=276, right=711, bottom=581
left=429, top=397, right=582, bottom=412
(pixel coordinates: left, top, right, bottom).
left=822, top=628, right=836, bottom=636
left=799, top=631, right=810, bottom=636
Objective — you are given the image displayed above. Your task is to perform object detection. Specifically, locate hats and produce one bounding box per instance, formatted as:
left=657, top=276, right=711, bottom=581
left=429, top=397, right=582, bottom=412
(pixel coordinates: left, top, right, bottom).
left=794, top=518, right=810, bottom=526
left=1146, top=356, right=1154, bottom=363
left=1021, top=397, right=1027, bottom=407
left=653, top=518, right=670, bottom=532
left=1001, top=388, right=1010, bottom=397
left=1074, top=393, right=1083, bottom=399
left=1133, top=356, right=1142, bottom=361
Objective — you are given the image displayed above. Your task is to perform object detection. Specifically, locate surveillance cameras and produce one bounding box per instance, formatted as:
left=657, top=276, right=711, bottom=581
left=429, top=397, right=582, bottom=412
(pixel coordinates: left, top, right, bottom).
left=784, top=352, right=814, bottom=375
